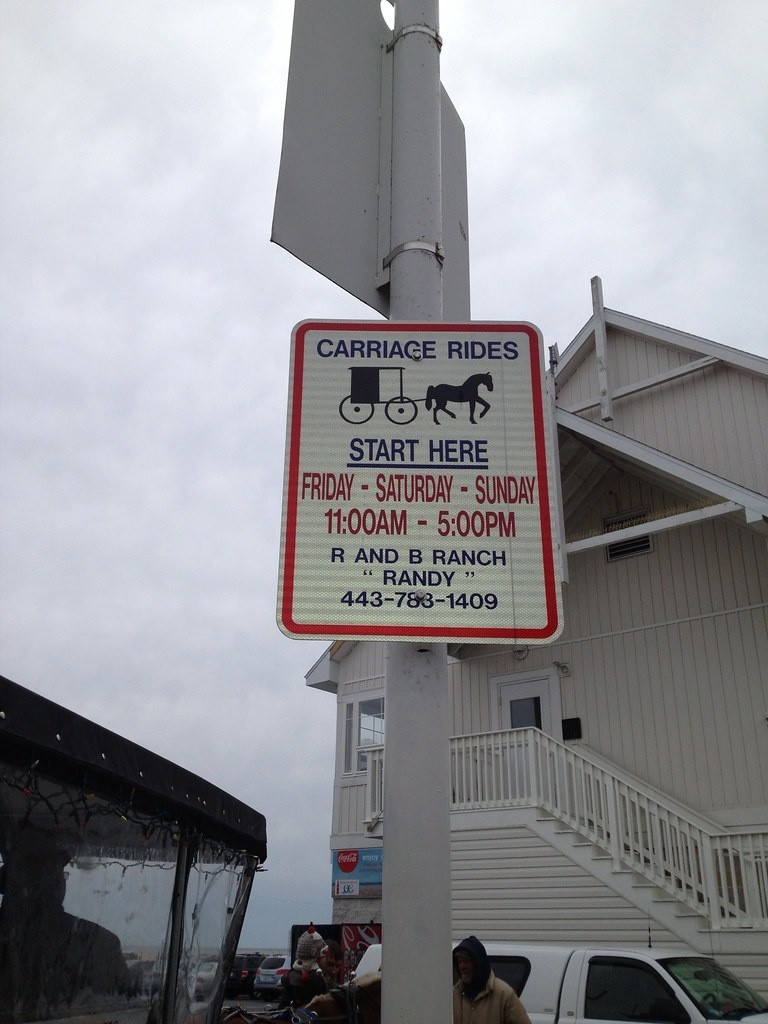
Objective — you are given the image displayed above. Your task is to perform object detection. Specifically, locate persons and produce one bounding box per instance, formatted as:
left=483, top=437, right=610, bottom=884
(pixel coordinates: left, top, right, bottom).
left=453, top=934, right=533, bottom=1024
left=0, top=819, right=134, bottom=1024
left=278, top=927, right=344, bottom=1011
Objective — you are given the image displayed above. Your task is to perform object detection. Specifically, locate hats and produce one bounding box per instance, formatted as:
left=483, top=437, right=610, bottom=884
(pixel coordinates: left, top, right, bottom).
left=296, top=926, right=328, bottom=982
left=454, top=947, right=473, bottom=961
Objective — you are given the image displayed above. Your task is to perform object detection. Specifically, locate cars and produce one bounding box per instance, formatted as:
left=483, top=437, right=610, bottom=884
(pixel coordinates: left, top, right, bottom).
left=123, top=959, right=221, bottom=1001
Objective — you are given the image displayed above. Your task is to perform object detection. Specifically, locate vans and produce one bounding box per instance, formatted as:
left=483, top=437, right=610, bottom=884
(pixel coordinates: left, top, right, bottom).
left=350, top=939, right=768, bottom=1024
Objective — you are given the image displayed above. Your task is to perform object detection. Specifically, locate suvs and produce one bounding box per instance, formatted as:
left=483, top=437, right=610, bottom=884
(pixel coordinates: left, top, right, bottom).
left=224, top=951, right=269, bottom=1001
left=254, top=953, right=292, bottom=1002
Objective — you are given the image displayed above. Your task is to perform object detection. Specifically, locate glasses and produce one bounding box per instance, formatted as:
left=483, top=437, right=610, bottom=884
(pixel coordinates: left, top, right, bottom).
left=60, top=871, right=71, bottom=880
